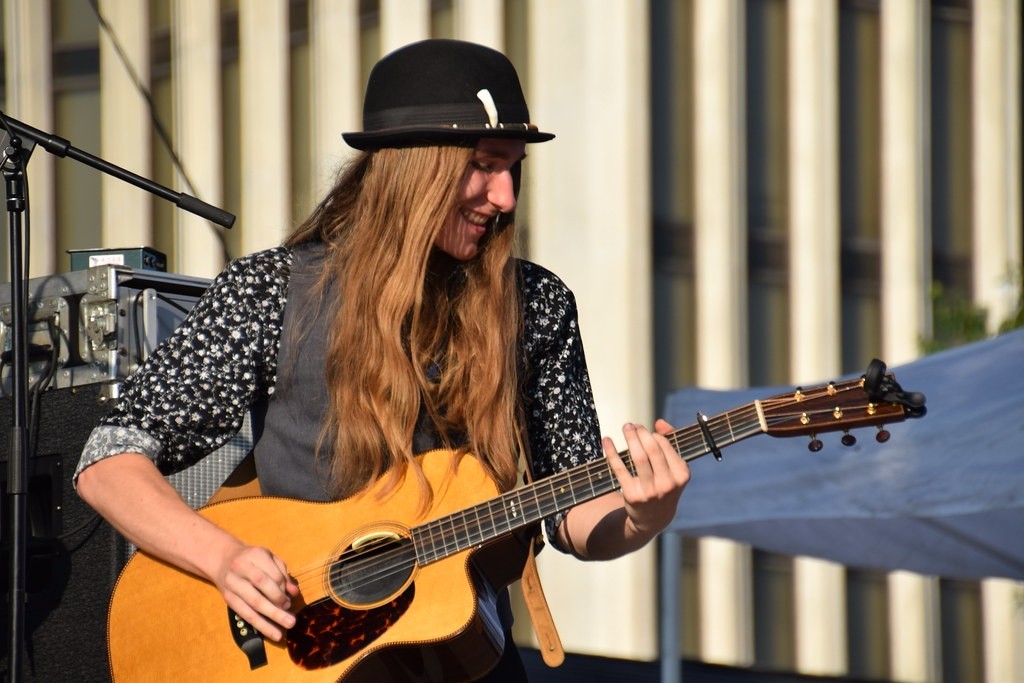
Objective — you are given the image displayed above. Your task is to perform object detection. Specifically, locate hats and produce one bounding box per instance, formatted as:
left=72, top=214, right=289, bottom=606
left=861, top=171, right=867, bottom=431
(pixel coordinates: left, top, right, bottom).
left=340, top=39, right=554, bottom=152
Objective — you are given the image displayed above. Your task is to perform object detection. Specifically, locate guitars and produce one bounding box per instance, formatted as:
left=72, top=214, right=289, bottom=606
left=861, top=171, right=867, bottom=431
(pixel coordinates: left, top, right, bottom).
left=104, top=357, right=928, bottom=683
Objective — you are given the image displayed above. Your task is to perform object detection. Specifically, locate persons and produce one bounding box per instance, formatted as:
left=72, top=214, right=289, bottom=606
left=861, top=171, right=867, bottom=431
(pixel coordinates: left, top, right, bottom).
left=67, top=37, right=692, bottom=683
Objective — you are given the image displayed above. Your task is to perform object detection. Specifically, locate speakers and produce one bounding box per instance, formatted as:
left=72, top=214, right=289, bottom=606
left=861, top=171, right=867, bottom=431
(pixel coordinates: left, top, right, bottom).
left=0, top=377, right=256, bottom=683
